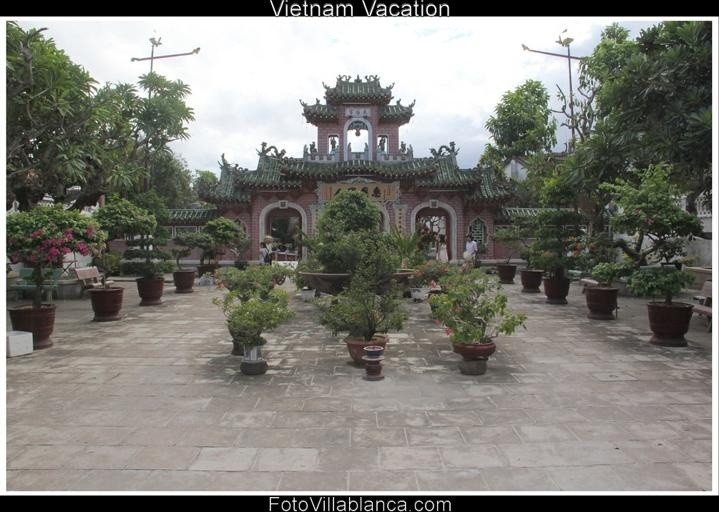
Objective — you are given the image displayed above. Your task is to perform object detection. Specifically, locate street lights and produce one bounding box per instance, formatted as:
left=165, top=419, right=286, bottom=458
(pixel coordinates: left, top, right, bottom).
left=519, top=29, right=595, bottom=156
left=130, top=35, right=203, bottom=197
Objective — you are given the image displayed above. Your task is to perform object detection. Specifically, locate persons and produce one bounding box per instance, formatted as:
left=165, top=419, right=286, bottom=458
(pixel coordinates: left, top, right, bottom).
left=464, top=234, right=478, bottom=268
left=258, top=242, right=271, bottom=266
left=437, top=234, right=448, bottom=264
